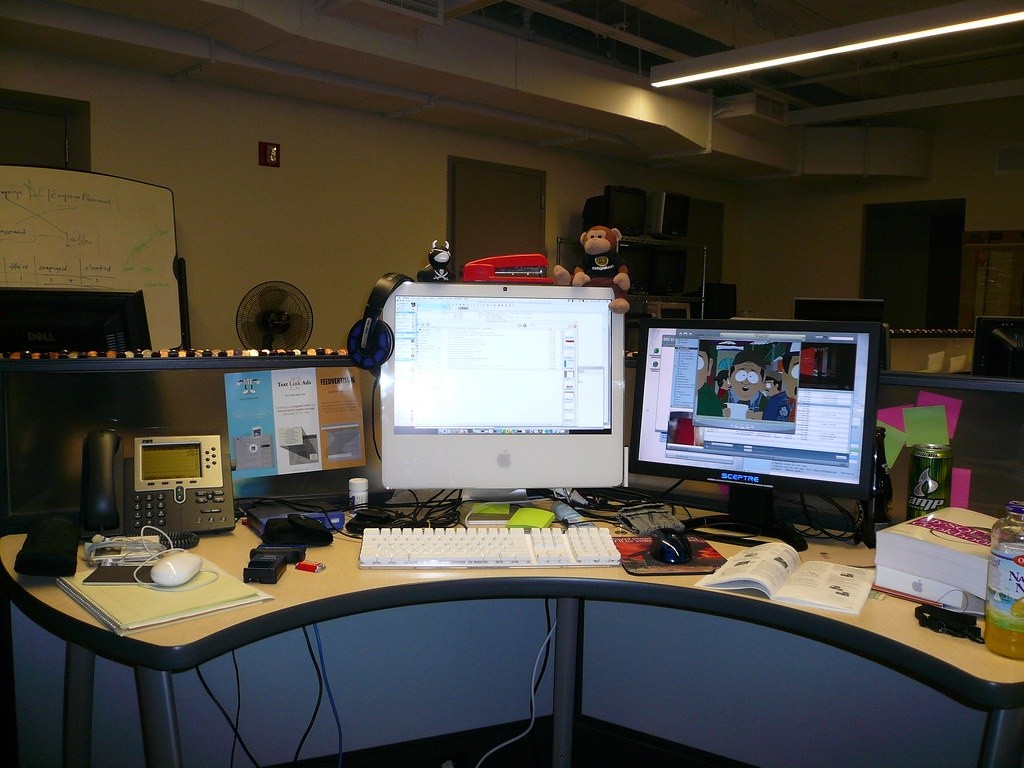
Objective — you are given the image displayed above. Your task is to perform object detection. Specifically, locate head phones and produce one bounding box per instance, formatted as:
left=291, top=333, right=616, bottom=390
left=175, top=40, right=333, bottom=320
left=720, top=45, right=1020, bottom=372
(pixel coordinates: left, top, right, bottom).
left=347, top=272, right=415, bottom=370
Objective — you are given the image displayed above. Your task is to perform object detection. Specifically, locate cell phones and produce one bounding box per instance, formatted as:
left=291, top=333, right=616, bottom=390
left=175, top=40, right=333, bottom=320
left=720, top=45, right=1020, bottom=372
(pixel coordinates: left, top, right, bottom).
left=89, top=547, right=122, bottom=564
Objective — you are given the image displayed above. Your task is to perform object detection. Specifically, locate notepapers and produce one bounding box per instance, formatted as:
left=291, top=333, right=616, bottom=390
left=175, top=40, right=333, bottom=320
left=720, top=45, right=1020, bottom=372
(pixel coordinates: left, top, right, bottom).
left=504, top=507, right=557, bottom=528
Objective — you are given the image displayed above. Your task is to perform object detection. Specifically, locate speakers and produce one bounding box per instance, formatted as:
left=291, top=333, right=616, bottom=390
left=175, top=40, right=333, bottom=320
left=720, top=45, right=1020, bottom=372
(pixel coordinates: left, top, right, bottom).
left=697, top=284, right=737, bottom=319
left=646, top=192, right=691, bottom=240
left=641, top=264, right=680, bottom=296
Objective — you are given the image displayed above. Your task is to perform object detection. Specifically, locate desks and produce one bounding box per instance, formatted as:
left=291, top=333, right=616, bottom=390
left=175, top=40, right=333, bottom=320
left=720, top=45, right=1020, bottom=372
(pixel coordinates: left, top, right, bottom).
left=0, top=498, right=1024, bottom=768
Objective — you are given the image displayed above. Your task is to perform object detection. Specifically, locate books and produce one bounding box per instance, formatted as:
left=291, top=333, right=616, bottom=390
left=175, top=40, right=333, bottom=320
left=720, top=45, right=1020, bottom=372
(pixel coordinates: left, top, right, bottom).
left=873, top=506, right=999, bottom=600
left=507, top=507, right=556, bottom=529
left=59, top=558, right=272, bottom=636
left=697, top=542, right=876, bottom=614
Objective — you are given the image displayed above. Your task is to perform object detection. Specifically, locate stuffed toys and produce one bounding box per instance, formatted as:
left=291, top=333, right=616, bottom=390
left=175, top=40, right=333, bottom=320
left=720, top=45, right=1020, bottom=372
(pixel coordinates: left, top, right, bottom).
left=553, top=226, right=631, bottom=313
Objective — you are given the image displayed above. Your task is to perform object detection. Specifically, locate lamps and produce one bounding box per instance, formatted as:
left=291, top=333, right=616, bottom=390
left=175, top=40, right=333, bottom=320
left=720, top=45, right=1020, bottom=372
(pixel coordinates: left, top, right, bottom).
left=649, top=0, right=1024, bottom=88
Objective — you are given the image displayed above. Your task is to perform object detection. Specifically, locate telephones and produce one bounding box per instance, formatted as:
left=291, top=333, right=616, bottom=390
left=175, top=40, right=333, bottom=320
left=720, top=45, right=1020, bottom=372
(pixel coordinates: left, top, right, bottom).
left=79, top=428, right=236, bottom=544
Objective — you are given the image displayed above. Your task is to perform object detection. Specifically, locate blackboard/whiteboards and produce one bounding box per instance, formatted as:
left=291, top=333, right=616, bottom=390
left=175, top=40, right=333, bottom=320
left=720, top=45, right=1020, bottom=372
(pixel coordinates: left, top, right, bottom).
left=0, top=164, right=181, bottom=352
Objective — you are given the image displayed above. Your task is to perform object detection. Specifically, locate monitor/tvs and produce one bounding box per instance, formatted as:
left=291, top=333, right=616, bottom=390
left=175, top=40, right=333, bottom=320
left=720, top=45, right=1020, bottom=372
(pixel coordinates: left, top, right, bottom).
left=627, top=317, right=883, bottom=552
left=971, top=315, right=1024, bottom=381
left=0, top=286, right=152, bottom=359
left=381, top=281, right=624, bottom=527
left=601, top=185, right=647, bottom=237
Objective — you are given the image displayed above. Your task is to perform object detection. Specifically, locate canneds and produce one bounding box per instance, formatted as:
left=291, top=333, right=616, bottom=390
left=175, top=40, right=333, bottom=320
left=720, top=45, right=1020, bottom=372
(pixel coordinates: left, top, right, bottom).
left=906, top=442, right=952, bottom=523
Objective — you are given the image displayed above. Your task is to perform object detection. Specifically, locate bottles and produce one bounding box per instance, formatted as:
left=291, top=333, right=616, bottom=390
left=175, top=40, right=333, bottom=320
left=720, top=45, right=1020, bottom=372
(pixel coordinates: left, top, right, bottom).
left=983, top=500, right=1024, bottom=659
left=854, top=426, right=893, bottom=548
left=349, top=479, right=368, bottom=516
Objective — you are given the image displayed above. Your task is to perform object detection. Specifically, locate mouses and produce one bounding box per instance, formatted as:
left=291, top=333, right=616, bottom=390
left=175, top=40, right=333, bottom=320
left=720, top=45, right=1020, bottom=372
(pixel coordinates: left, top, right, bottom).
left=648, top=529, right=691, bottom=563
left=151, top=553, right=203, bottom=587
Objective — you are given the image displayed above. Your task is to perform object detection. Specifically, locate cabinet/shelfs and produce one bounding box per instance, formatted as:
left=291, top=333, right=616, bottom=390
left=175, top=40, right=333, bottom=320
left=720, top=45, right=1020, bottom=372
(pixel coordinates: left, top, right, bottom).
left=558, top=236, right=708, bottom=362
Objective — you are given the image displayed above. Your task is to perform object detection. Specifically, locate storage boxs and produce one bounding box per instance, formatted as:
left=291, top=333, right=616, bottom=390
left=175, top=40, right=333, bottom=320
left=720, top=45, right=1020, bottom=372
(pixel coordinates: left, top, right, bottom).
left=877, top=564, right=985, bottom=612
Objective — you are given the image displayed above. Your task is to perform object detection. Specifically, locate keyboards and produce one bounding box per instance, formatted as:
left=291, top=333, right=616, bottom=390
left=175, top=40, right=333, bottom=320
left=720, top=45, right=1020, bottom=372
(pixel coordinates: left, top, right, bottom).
left=357, top=527, right=622, bottom=568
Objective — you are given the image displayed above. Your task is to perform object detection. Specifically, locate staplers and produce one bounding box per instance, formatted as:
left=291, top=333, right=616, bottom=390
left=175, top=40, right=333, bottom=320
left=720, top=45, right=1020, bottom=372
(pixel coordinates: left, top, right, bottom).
left=463, top=254, right=555, bottom=284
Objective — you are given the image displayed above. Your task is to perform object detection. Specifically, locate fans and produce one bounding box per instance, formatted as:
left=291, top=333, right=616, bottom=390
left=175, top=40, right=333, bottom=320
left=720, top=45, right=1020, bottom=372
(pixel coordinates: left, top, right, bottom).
left=235, top=282, right=314, bottom=351
